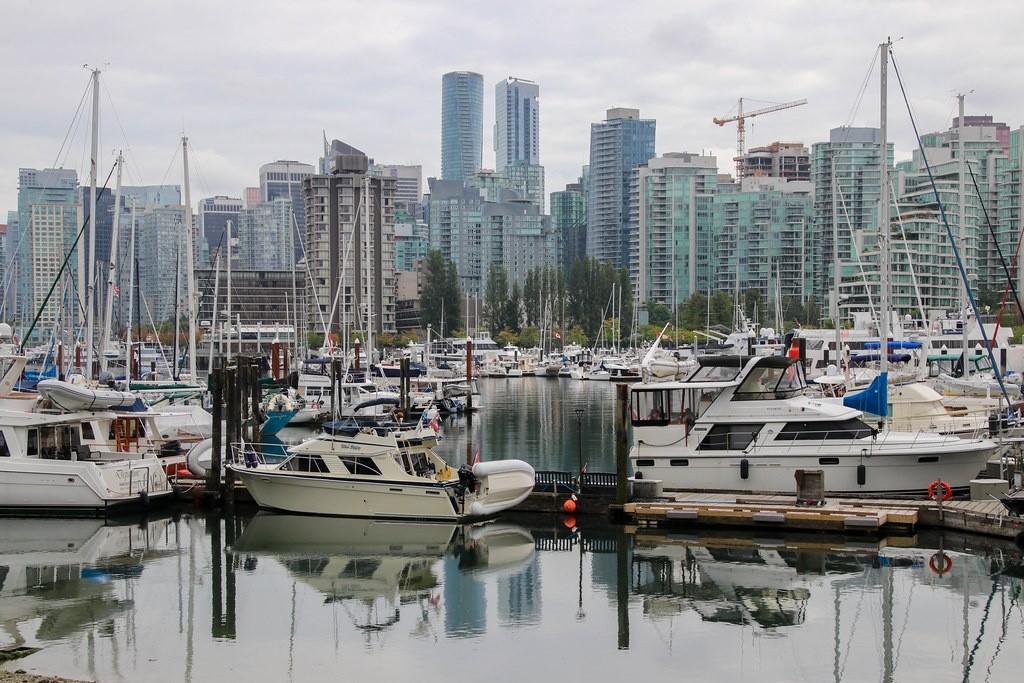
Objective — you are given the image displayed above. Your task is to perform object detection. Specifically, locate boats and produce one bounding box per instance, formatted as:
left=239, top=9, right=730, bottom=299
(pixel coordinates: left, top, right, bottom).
left=0, top=511, right=178, bottom=591
left=631, top=544, right=980, bottom=641
left=224, top=507, right=535, bottom=632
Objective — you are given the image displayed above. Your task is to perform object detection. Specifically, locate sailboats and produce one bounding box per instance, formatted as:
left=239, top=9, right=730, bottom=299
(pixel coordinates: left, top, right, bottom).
left=0, top=62, right=1023, bottom=526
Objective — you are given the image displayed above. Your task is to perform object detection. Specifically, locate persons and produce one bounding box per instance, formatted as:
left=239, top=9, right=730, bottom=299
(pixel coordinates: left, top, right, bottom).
left=682, top=408, right=697, bottom=432
left=475, top=358, right=481, bottom=374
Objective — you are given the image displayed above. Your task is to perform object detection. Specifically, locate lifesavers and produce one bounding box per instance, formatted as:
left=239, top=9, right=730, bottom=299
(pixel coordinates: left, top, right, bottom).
left=929, top=481, right=950, bottom=500
left=347, top=374, right=352, bottom=382
left=930, top=554, right=952, bottom=573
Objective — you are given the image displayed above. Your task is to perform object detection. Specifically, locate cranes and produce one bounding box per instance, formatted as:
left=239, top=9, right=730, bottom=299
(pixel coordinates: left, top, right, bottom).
left=713, top=96, right=808, bottom=185
